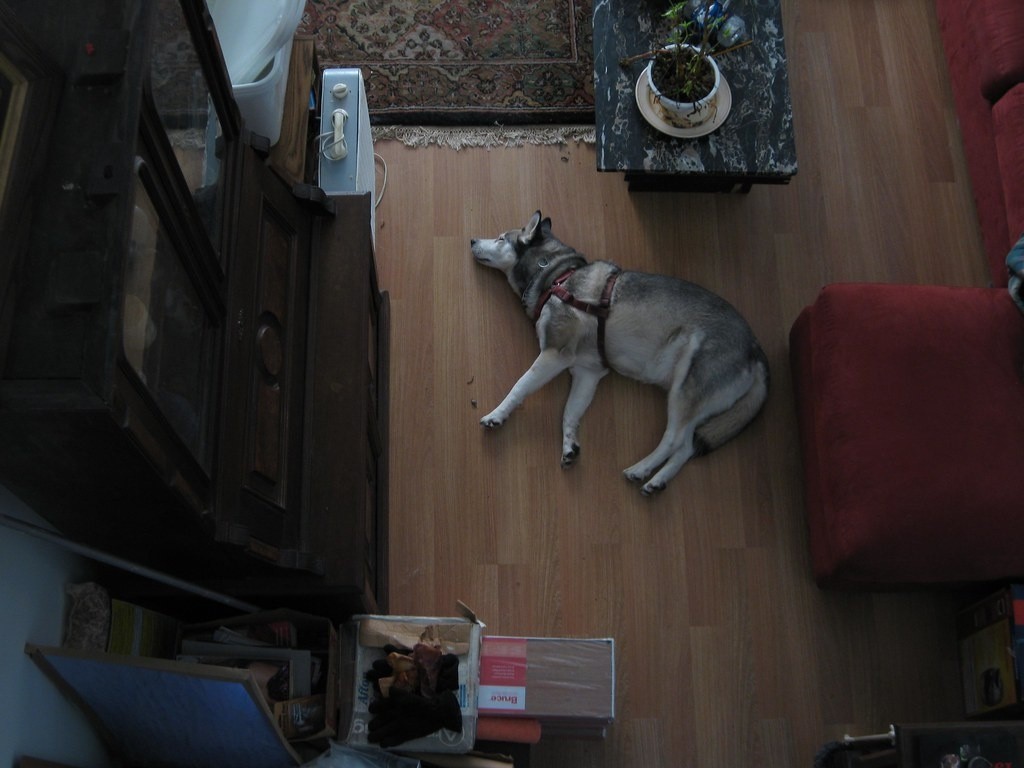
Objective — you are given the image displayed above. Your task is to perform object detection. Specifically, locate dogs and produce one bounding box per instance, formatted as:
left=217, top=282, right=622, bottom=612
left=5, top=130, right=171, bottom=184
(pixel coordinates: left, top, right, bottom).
left=468, top=208, right=772, bottom=499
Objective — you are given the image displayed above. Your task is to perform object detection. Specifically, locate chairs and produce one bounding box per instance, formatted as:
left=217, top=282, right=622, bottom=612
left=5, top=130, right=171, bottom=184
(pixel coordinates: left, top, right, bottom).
left=25, top=641, right=305, bottom=768
left=812, top=723, right=897, bottom=768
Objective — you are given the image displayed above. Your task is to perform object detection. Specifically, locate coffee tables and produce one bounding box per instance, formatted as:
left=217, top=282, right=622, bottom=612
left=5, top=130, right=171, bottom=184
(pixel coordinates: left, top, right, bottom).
left=592, top=0, right=798, bottom=193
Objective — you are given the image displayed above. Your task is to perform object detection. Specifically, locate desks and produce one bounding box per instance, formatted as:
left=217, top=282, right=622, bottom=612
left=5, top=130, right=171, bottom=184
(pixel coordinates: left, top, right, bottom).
left=898, top=719, right=1024, bottom=768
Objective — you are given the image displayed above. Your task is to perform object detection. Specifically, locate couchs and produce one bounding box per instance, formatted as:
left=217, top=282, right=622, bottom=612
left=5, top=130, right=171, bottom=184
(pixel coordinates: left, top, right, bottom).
left=788, top=0, right=1023, bottom=594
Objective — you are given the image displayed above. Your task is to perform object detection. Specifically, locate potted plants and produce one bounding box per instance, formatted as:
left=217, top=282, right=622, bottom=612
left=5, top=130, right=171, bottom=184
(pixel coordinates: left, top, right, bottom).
left=646, top=0, right=752, bottom=125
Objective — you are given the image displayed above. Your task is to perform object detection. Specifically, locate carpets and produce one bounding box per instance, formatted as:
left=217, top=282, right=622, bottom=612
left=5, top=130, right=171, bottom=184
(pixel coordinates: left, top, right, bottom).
left=296, top=1, right=598, bottom=152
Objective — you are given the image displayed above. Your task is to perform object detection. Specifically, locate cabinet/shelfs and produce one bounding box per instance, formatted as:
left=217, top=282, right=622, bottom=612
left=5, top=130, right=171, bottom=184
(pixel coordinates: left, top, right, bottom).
left=0, top=0, right=391, bottom=621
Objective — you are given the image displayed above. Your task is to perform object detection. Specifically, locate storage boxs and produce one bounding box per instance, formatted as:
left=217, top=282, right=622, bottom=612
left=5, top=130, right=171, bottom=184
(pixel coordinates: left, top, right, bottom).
left=205, top=0, right=305, bottom=148
left=179, top=601, right=616, bottom=754
left=958, top=584, right=1024, bottom=717
